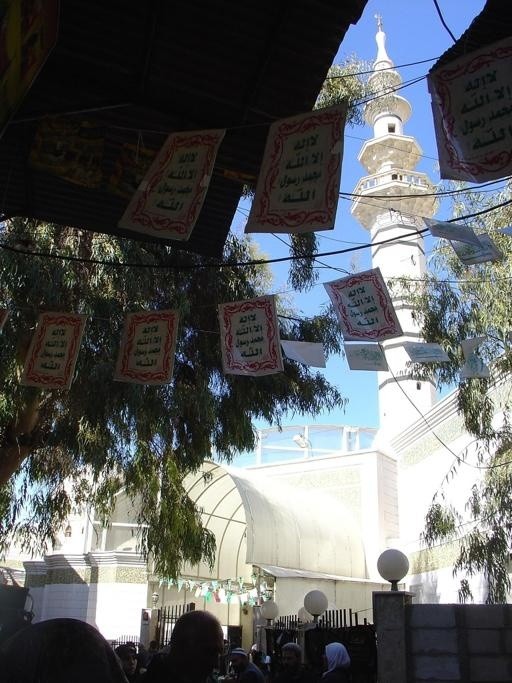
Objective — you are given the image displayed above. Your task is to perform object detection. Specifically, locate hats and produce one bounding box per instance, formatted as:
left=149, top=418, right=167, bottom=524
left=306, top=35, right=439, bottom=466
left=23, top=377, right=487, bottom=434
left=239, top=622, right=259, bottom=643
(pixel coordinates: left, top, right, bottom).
left=230, top=648, right=248, bottom=657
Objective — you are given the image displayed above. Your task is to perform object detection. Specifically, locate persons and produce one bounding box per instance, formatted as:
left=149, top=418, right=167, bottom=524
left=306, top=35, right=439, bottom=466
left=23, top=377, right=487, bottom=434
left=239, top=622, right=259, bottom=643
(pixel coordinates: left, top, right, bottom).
left=1, top=609, right=354, bottom=682
